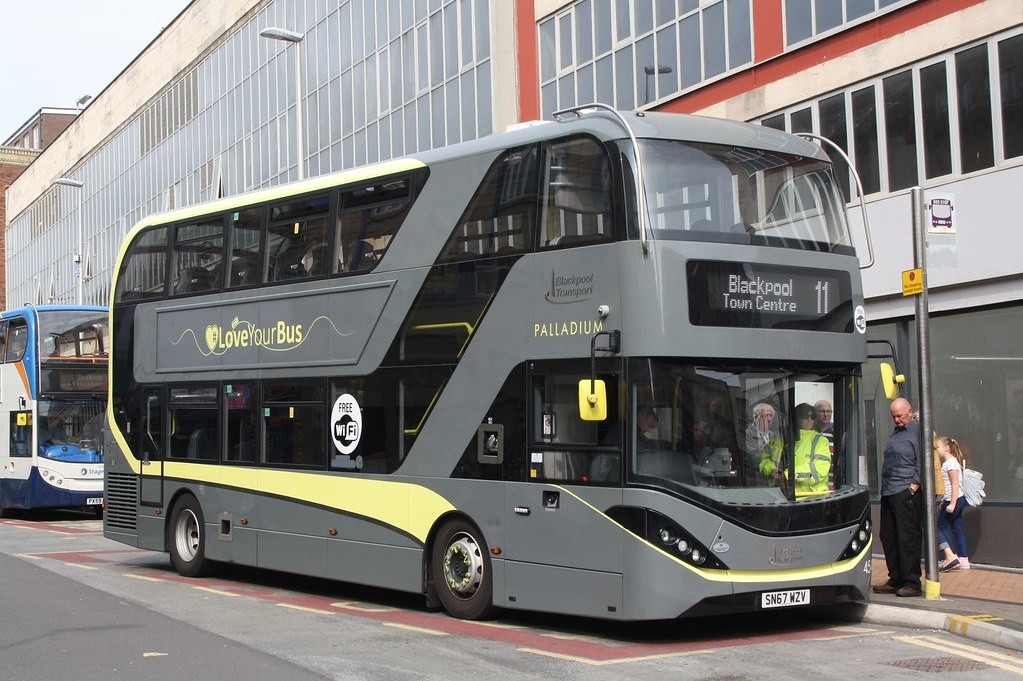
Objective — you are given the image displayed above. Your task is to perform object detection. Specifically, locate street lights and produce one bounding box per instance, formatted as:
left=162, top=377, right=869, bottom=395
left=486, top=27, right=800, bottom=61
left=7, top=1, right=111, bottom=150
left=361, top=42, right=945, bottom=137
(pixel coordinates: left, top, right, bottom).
left=259, top=27, right=306, bottom=182
left=53, top=178, right=84, bottom=356
left=642, top=64, right=673, bottom=104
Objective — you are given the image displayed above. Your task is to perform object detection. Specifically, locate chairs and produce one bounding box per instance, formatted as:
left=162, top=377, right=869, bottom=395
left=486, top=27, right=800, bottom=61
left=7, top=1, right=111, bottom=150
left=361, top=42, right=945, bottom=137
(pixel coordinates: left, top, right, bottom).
left=17, top=349, right=109, bottom=362
left=308, top=246, right=342, bottom=276
left=276, top=253, right=307, bottom=279
left=175, top=267, right=210, bottom=295
left=240, top=258, right=274, bottom=283
left=212, top=264, right=242, bottom=288
left=346, top=241, right=378, bottom=272
left=449, top=233, right=612, bottom=257
left=187, top=429, right=219, bottom=459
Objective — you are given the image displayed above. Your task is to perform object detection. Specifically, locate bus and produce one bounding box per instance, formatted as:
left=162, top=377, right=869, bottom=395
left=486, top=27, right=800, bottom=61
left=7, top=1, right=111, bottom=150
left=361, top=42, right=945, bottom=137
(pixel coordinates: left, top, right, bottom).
left=102, top=104, right=905, bottom=623
left=0, top=302, right=109, bottom=519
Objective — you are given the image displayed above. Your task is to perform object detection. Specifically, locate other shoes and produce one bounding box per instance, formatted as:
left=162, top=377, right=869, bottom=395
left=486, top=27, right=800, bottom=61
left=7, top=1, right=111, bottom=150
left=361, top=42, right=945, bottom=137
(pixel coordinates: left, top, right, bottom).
left=938, top=559, right=959, bottom=572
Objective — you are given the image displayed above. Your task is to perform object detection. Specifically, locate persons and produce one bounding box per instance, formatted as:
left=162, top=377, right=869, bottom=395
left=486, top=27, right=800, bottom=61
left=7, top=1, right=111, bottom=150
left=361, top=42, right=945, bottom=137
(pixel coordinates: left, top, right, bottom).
left=600, top=402, right=659, bottom=473
left=39, top=424, right=79, bottom=451
left=872, top=399, right=971, bottom=599
left=744, top=402, right=840, bottom=498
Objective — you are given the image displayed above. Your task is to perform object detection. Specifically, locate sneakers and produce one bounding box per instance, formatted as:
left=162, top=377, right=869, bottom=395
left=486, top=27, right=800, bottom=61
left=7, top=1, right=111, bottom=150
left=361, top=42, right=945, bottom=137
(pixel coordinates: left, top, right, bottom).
left=897, top=585, right=922, bottom=597
left=958, top=557, right=971, bottom=569
left=874, top=583, right=897, bottom=594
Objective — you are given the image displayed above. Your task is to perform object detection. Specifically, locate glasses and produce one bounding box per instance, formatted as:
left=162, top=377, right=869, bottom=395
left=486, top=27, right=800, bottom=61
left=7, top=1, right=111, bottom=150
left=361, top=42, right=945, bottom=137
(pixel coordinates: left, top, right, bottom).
left=802, top=414, right=816, bottom=420
left=819, top=410, right=832, bottom=414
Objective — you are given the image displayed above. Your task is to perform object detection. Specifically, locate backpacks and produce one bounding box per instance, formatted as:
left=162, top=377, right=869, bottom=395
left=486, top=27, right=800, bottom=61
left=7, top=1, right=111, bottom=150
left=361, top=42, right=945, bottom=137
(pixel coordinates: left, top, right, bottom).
left=952, top=460, right=986, bottom=507
